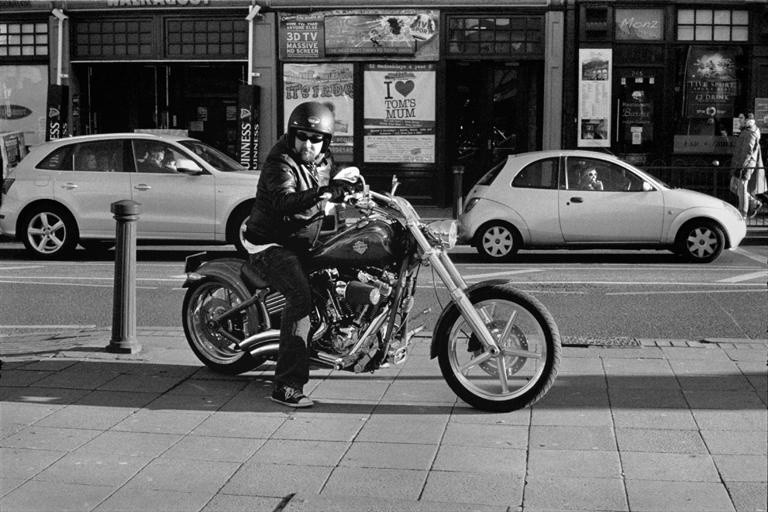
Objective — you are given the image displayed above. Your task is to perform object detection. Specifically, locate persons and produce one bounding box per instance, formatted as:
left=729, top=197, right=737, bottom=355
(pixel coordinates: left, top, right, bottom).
left=579, top=166, right=604, bottom=190
left=729, top=109, right=768, bottom=221
left=80, top=143, right=177, bottom=172
left=245, top=102, right=355, bottom=407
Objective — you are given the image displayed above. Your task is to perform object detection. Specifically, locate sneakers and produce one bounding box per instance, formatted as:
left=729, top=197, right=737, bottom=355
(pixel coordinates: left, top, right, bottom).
left=749, top=201, right=762, bottom=218
left=269, top=385, right=313, bottom=409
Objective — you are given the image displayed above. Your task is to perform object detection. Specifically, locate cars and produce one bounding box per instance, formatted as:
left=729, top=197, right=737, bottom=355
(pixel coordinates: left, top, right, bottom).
left=458, top=149, right=747, bottom=264
left=0, top=132, right=263, bottom=261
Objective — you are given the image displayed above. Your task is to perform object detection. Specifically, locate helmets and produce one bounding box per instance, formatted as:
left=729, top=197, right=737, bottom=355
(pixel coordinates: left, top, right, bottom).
left=289, top=101, right=333, bottom=153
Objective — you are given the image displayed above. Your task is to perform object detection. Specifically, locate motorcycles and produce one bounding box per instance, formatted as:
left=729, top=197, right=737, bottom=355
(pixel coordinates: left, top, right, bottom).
left=182, top=165, right=562, bottom=414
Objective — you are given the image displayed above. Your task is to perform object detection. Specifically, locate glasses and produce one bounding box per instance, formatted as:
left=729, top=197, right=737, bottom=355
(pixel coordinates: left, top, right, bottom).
left=294, top=129, right=325, bottom=144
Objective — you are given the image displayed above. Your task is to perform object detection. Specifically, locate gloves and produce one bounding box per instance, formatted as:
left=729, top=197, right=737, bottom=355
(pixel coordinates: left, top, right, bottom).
left=318, top=184, right=343, bottom=204
